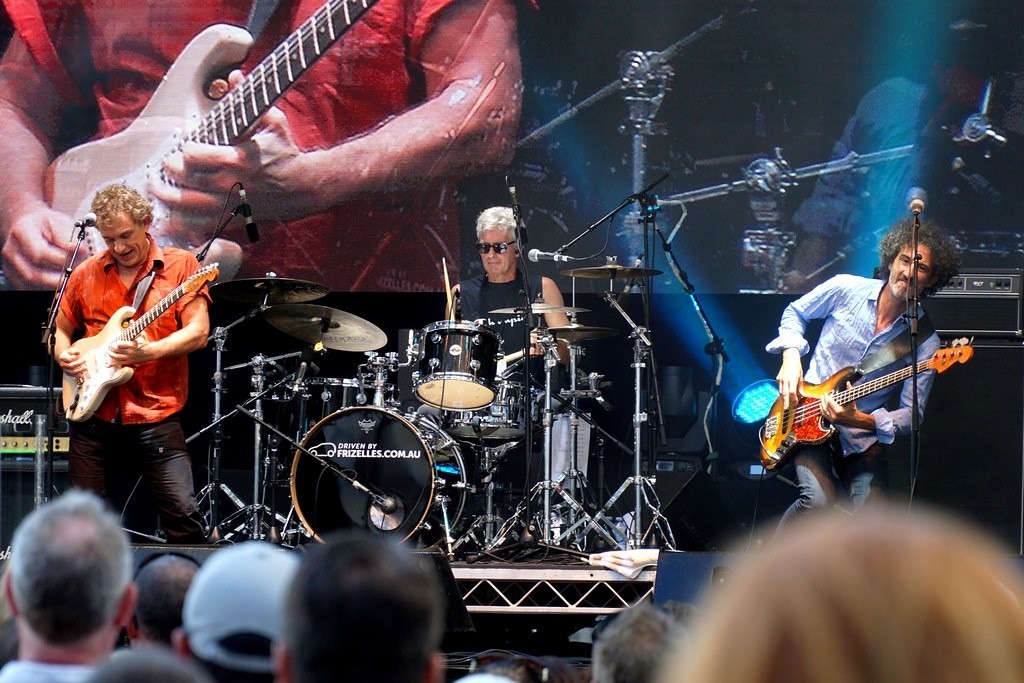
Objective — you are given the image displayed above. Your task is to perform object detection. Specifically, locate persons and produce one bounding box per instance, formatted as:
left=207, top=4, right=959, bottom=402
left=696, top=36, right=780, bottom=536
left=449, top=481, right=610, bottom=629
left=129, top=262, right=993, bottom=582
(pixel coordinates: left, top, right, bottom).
left=447, top=205, right=570, bottom=538
left=127, top=555, right=197, bottom=649
left=48, top=185, right=213, bottom=543
left=86, top=646, right=210, bottom=683
left=787, top=56, right=1007, bottom=296
left=171, top=541, right=301, bottom=683
left=0, top=0, right=520, bottom=292
left=0, top=491, right=132, bottom=682
left=766, top=217, right=958, bottom=539
left=272, top=535, right=514, bottom=683
left=592, top=601, right=695, bottom=683
left=645, top=505, right=1024, bottom=682
left=479, top=655, right=592, bottom=683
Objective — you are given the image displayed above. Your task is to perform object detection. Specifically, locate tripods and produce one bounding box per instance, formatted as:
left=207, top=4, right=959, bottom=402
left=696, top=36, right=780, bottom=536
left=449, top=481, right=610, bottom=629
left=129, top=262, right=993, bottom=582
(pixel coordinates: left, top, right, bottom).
left=186, top=195, right=668, bottom=564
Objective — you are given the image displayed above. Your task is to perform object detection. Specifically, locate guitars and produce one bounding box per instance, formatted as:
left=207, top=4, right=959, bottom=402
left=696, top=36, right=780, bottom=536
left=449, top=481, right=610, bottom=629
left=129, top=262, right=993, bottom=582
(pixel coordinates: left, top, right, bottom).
left=61, top=261, right=221, bottom=422
left=761, top=334, right=973, bottom=474
left=43, top=0, right=375, bottom=287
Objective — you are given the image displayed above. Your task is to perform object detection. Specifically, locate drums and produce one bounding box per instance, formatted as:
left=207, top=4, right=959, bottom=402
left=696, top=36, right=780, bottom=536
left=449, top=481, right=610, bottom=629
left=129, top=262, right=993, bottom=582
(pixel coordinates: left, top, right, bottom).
left=446, top=380, right=527, bottom=439
left=289, top=404, right=468, bottom=553
left=271, top=377, right=402, bottom=487
left=407, top=317, right=505, bottom=411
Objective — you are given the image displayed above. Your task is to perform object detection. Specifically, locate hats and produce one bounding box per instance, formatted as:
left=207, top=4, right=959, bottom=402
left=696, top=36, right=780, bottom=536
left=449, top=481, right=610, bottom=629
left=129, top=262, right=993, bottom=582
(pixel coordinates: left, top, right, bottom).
left=186, top=541, right=300, bottom=670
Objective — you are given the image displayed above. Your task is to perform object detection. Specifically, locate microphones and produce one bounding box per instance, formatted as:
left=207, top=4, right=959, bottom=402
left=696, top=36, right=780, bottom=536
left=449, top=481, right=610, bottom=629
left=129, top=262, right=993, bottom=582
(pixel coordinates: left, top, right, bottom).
left=510, top=185, right=528, bottom=245
left=379, top=494, right=398, bottom=515
left=501, top=355, right=533, bottom=378
left=238, top=182, right=261, bottom=242
left=906, top=187, right=929, bottom=214
left=527, top=249, right=575, bottom=263
left=617, top=254, right=642, bottom=310
left=262, top=354, right=288, bottom=376
left=74, top=212, right=97, bottom=228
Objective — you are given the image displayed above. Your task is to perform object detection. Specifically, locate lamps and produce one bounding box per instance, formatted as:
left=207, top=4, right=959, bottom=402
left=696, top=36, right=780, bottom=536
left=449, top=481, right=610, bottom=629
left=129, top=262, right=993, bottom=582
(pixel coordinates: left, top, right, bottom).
left=731, top=378, right=781, bottom=452
left=657, top=367, right=699, bottom=440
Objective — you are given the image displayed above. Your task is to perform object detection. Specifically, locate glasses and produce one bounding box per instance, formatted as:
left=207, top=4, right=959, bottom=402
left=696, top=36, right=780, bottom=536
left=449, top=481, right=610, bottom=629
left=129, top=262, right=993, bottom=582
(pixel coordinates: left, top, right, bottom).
left=475, top=238, right=516, bottom=253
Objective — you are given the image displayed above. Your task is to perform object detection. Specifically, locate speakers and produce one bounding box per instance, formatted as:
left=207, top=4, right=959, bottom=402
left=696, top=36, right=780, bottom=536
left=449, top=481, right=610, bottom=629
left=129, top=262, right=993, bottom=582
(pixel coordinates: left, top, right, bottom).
left=653, top=551, right=739, bottom=613
left=0, top=461, right=70, bottom=565
left=883, top=339, right=1024, bottom=558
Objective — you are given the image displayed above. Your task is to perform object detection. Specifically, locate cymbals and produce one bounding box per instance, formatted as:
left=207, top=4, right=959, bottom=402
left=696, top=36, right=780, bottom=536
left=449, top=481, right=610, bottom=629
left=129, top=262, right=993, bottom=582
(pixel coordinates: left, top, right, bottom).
left=547, top=318, right=619, bottom=348
left=487, top=300, right=592, bottom=314
left=208, top=275, right=330, bottom=303
left=263, top=304, right=388, bottom=352
left=559, top=264, right=664, bottom=278
